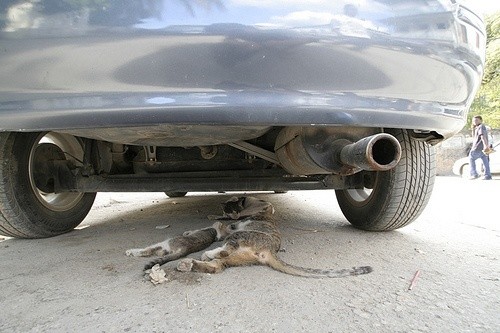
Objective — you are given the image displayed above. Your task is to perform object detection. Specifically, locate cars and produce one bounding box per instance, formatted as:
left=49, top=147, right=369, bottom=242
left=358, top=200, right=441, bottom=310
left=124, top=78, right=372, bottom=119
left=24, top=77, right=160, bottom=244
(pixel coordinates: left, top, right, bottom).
left=0, top=0, right=486, bottom=238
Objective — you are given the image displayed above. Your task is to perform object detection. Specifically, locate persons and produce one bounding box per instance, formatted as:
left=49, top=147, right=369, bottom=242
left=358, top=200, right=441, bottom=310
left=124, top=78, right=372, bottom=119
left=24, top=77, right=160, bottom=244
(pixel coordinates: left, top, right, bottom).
left=468, top=115, right=493, bottom=180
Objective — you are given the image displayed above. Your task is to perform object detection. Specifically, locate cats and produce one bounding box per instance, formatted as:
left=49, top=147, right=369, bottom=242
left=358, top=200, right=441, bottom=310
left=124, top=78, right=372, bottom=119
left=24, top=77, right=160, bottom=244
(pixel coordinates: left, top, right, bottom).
left=176, top=220, right=373, bottom=279
left=207, top=195, right=275, bottom=220
left=126, top=221, right=226, bottom=271
